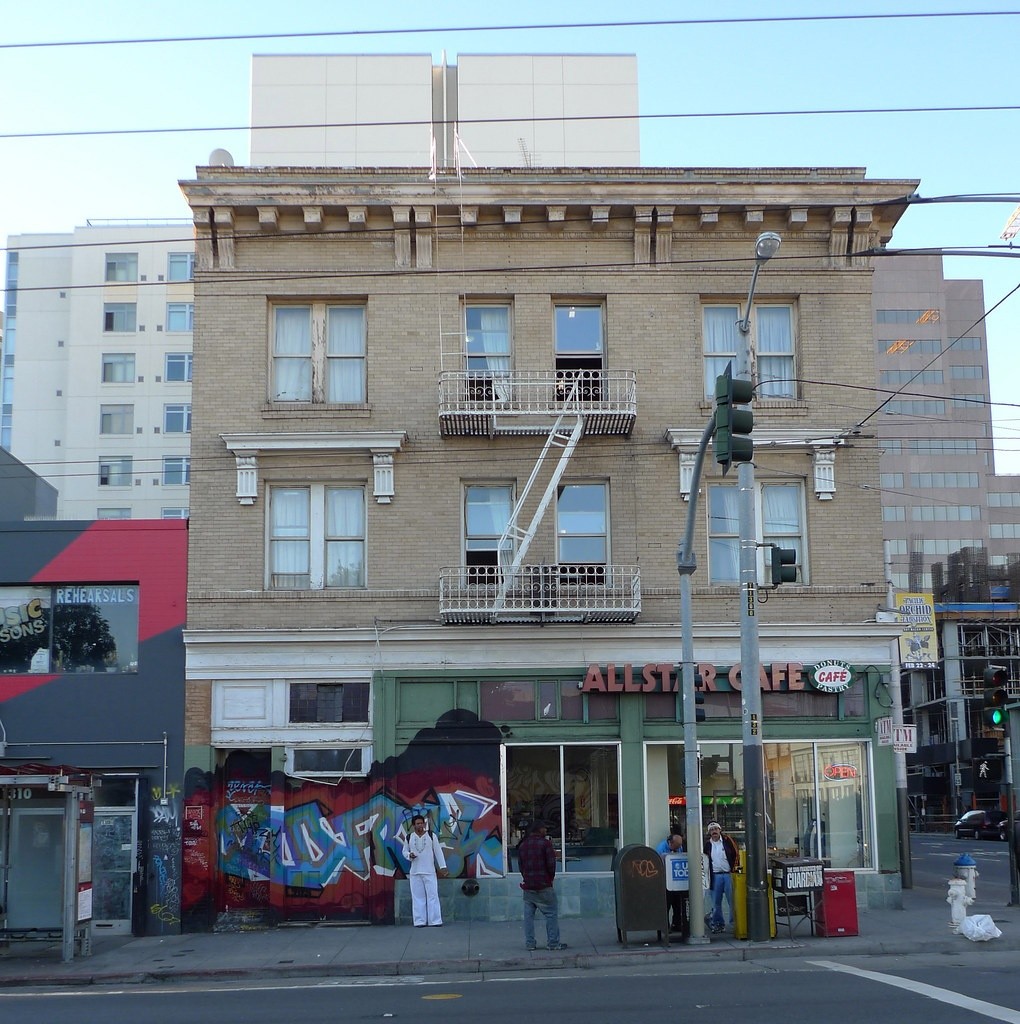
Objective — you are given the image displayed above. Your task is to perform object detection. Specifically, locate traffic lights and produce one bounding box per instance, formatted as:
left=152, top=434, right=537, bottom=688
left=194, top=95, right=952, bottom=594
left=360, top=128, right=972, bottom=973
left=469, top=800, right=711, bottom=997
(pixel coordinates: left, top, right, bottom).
left=982, top=667, right=1010, bottom=727
left=714, top=359, right=754, bottom=478
left=772, top=543, right=797, bottom=584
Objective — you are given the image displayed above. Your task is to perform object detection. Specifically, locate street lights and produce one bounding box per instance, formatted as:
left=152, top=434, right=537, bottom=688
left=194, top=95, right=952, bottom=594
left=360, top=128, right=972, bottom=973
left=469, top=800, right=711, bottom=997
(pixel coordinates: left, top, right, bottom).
left=734, top=231, right=782, bottom=942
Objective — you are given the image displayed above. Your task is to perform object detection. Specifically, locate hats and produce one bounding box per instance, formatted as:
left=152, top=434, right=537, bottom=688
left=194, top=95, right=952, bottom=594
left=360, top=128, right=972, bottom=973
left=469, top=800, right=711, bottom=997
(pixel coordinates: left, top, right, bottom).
left=707, top=822, right=721, bottom=832
left=532, top=820, right=549, bottom=831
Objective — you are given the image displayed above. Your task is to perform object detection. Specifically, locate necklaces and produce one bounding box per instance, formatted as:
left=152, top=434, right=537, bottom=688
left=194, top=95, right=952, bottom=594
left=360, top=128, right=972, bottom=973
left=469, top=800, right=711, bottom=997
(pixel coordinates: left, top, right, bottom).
left=412, top=830, right=427, bottom=853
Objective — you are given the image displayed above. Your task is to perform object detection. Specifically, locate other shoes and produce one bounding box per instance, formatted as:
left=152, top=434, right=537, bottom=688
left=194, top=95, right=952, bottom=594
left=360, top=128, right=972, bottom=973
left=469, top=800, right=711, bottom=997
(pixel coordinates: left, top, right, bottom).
left=546, top=943, right=567, bottom=951
left=527, top=946, right=536, bottom=951
left=669, top=929, right=672, bottom=934
left=671, top=924, right=681, bottom=930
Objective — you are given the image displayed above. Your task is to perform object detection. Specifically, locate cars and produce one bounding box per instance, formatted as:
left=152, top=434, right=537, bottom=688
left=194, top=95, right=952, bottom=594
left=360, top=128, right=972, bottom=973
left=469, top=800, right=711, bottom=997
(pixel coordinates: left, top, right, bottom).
left=997, top=810, right=1020, bottom=841
left=953, top=809, right=1007, bottom=840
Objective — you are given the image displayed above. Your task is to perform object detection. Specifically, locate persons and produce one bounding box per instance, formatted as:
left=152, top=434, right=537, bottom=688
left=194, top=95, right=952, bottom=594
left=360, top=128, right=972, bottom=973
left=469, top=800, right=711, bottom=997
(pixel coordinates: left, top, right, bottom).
left=655, top=833, right=691, bottom=932
left=704, top=821, right=742, bottom=932
left=517, top=819, right=568, bottom=951
left=402, top=815, right=448, bottom=928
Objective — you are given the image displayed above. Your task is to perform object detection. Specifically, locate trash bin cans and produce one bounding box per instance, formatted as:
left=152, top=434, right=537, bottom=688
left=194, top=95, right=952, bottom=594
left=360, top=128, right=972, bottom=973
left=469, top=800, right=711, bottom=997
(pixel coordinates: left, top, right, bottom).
left=613, top=844, right=670, bottom=947
left=814, top=870, right=858, bottom=937
